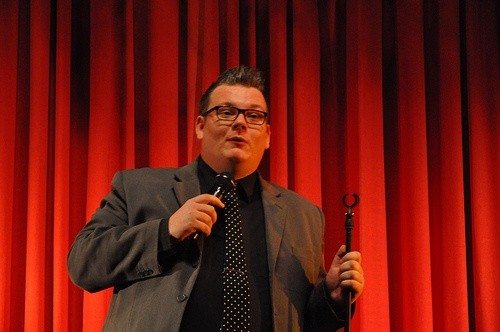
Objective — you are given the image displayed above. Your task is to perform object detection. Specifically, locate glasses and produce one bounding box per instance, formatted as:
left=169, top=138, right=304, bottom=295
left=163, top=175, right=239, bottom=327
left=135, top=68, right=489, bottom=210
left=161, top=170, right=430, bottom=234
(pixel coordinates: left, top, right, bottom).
left=202, top=105, right=270, bottom=125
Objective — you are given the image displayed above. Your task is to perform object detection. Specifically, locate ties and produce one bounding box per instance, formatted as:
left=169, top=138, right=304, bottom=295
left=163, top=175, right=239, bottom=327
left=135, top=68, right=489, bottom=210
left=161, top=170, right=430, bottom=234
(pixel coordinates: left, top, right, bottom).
left=219, top=182, right=251, bottom=332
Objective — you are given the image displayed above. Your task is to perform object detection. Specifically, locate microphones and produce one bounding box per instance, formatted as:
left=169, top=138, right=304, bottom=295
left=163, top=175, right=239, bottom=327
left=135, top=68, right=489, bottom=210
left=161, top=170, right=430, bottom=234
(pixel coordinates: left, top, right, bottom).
left=192, top=171, right=235, bottom=240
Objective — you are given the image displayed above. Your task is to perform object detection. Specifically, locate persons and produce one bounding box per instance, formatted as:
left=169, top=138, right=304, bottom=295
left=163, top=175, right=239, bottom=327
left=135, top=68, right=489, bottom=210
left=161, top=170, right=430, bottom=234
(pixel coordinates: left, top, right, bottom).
left=68, top=66, right=364, bottom=332
